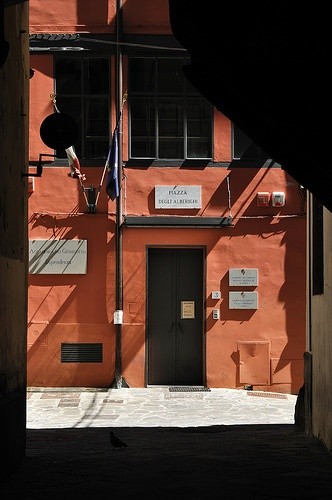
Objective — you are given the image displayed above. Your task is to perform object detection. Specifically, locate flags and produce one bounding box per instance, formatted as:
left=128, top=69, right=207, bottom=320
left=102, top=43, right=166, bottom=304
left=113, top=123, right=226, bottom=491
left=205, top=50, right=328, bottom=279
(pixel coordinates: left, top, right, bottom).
left=106, top=122, right=121, bottom=201
left=53, top=105, right=87, bottom=180
